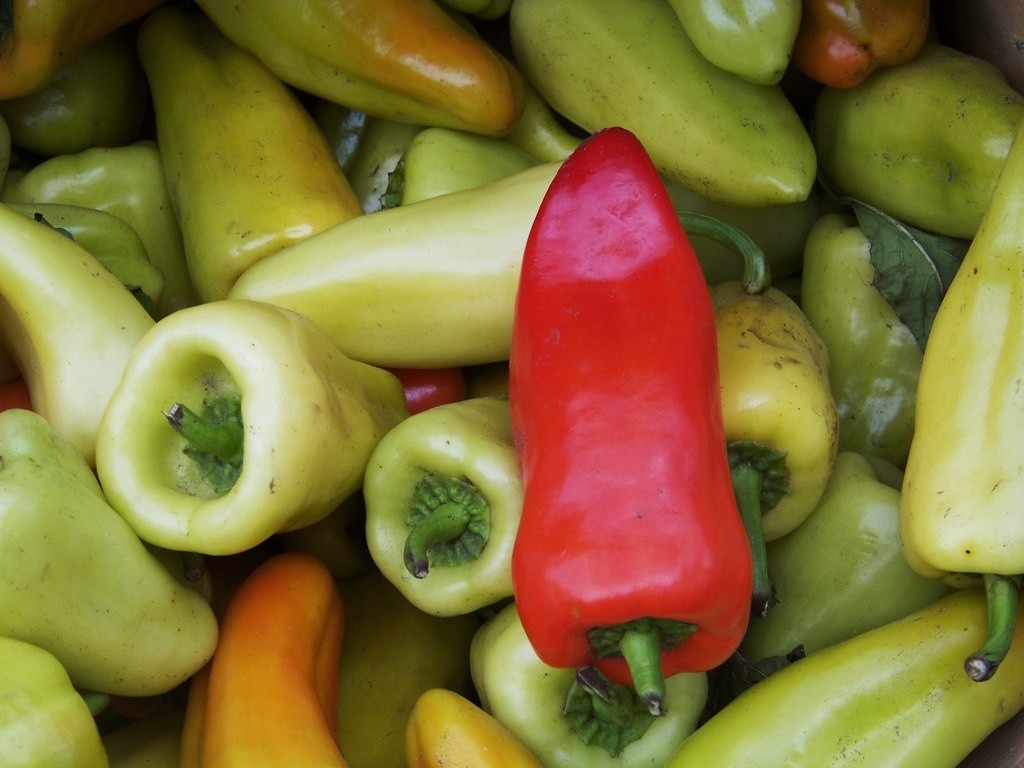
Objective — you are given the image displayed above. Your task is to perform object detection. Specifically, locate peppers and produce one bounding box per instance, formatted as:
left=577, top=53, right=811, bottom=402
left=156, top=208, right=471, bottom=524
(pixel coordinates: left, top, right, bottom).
left=0, top=0, right=1024, bottom=768
